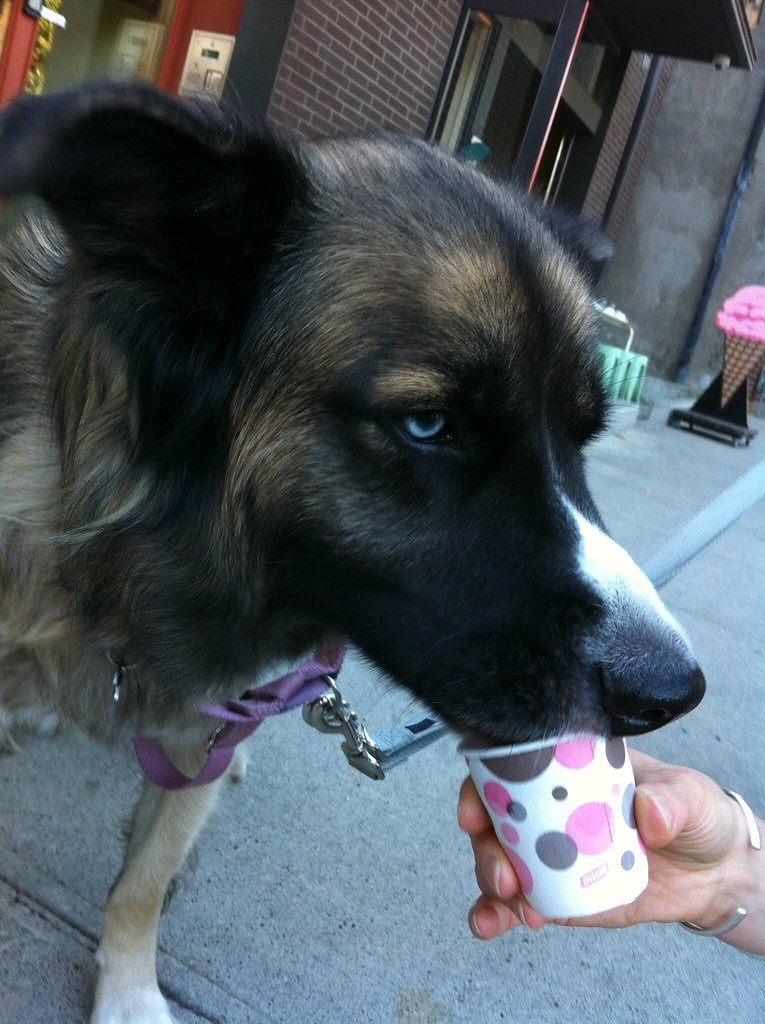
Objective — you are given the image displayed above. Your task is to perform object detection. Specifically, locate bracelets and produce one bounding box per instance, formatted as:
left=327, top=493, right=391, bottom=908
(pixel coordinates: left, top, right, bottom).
left=678, top=786, right=761, bottom=937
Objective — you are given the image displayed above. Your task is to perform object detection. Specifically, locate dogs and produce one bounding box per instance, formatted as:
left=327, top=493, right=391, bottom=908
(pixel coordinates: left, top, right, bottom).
left=1, top=75, right=707, bottom=1024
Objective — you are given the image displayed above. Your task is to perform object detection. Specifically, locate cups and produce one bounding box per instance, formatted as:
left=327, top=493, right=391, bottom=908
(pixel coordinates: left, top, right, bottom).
left=454, top=725, right=649, bottom=920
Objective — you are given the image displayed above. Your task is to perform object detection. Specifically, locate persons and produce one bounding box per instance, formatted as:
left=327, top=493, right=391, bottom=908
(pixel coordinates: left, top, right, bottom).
left=458, top=739, right=764, bottom=963
